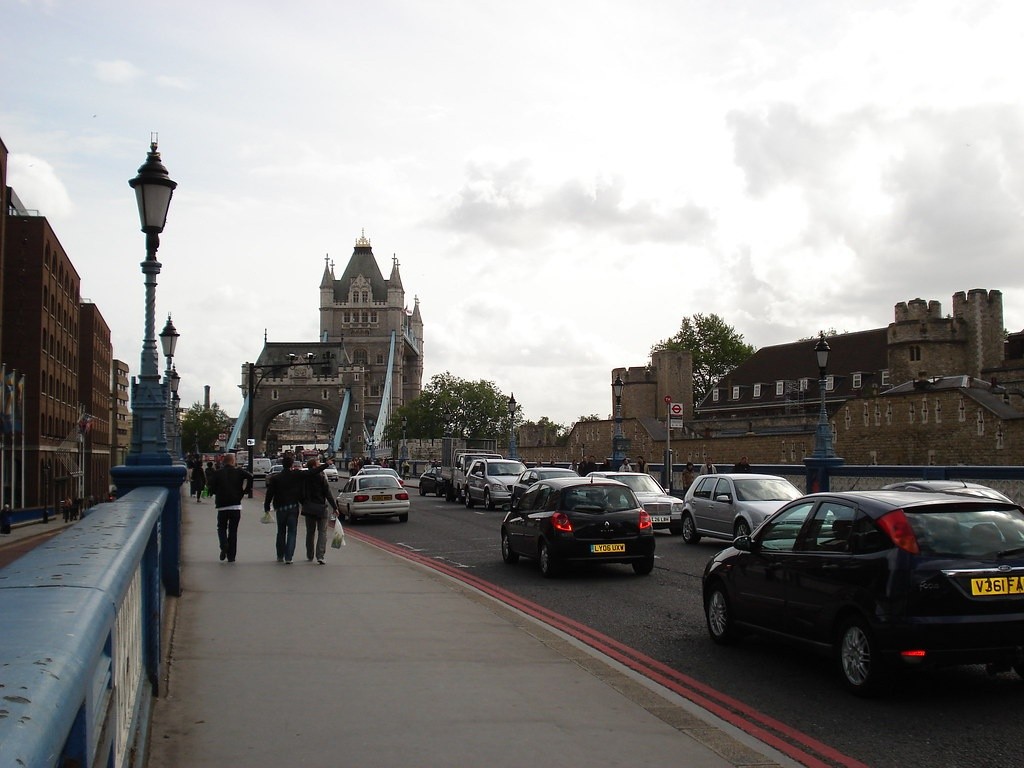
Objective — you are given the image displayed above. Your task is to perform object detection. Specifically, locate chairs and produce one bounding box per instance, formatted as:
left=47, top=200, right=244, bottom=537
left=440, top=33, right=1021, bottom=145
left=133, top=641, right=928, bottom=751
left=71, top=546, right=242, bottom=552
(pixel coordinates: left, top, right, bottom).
left=819, top=519, right=853, bottom=550
left=968, top=523, right=1001, bottom=546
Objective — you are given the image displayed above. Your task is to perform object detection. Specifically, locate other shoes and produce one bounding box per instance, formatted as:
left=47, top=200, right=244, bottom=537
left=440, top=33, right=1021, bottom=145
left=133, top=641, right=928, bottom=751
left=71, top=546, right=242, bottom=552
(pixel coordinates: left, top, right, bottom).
left=277, top=556, right=284, bottom=563
left=227, top=554, right=236, bottom=562
left=219, top=549, right=226, bottom=561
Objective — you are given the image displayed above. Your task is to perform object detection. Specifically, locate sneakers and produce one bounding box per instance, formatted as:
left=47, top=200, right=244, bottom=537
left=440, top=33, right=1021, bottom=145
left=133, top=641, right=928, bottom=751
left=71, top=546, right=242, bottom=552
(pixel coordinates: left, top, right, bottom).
left=317, top=557, right=327, bottom=565
left=286, top=559, right=294, bottom=564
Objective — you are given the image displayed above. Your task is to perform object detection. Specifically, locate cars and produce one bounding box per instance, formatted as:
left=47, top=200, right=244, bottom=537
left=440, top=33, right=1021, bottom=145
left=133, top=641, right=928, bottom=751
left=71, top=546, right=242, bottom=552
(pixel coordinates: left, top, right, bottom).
left=335, top=465, right=411, bottom=523
left=419, top=465, right=447, bottom=497
left=510, top=467, right=684, bottom=536
left=501, top=477, right=656, bottom=577
left=702, top=479, right=1024, bottom=696
left=252, top=457, right=339, bottom=482
left=680, top=473, right=837, bottom=544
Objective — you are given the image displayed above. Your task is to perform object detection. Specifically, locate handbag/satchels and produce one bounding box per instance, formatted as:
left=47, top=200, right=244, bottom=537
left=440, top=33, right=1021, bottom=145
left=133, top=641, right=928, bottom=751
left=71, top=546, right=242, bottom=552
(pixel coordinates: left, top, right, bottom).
left=330, top=518, right=346, bottom=550
left=258, top=512, right=275, bottom=524
left=200, top=486, right=208, bottom=499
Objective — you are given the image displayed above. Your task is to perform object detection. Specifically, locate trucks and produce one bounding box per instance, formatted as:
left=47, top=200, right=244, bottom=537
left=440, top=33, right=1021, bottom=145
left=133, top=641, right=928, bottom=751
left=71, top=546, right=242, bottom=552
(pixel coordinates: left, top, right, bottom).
left=440, top=449, right=503, bottom=504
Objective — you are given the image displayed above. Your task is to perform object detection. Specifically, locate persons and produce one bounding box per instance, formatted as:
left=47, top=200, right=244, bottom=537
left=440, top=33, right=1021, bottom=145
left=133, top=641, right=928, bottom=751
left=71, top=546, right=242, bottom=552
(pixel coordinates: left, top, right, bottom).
left=401, top=459, right=410, bottom=480
left=619, top=458, right=634, bottom=472
left=534, top=461, right=543, bottom=468
left=700, top=457, right=717, bottom=475
left=635, top=456, right=649, bottom=474
left=522, top=460, right=528, bottom=469
left=348, top=457, right=389, bottom=478
left=732, top=456, right=753, bottom=473
left=549, top=461, right=556, bottom=468
left=568, top=455, right=611, bottom=477
left=191, top=454, right=254, bottom=562
left=424, top=458, right=439, bottom=472
left=264, top=455, right=339, bottom=565
left=682, top=462, right=695, bottom=499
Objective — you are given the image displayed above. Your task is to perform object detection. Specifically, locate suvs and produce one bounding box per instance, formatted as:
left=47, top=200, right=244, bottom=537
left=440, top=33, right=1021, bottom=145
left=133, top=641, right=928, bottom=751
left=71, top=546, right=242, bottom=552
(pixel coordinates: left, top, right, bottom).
left=464, top=458, right=529, bottom=510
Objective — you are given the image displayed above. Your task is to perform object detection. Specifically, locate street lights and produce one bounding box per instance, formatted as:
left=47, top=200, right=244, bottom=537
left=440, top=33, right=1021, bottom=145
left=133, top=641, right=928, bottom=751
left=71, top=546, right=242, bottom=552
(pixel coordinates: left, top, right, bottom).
left=812, top=329, right=836, bottom=458
left=507, top=392, right=517, bottom=460
left=400, top=415, right=408, bottom=458
left=314, top=421, right=375, bottom=458
left=43, top=463, right=51, bottom=523
left=611, top=373, right=626, bottom=459
left=444, top=408, right=452, bottom=438
left=127, top=131, right=174, bottom=466
left=160, top=313, right=182, bottom=461
